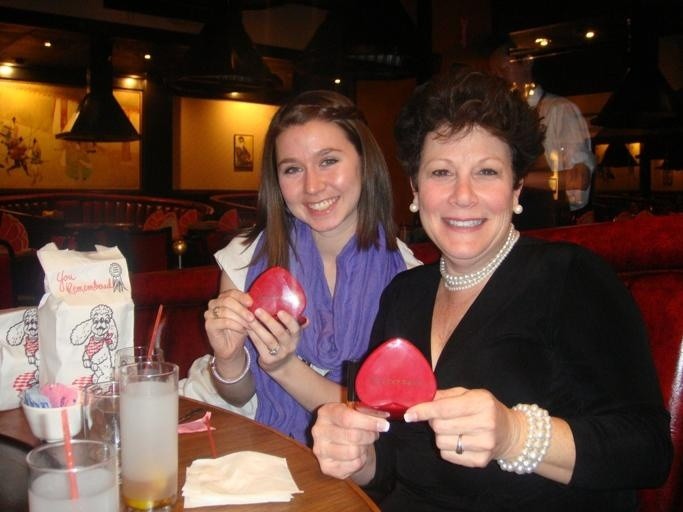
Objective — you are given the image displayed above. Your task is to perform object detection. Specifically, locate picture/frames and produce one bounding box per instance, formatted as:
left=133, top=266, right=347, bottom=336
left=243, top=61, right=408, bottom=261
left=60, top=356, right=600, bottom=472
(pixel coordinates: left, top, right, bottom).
left=232, top=134, right=256, bottom=172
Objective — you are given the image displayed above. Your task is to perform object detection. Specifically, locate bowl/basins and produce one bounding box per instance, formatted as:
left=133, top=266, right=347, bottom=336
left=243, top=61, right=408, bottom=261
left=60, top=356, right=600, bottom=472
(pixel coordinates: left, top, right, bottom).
left=21, top=400, right=83, bottom=443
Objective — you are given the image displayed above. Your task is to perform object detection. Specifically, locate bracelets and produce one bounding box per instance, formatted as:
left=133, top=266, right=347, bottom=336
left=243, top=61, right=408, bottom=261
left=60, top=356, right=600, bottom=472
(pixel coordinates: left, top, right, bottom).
left=209, top=346, right=251, bottom=385
left=499, top=400, right=552, bottom=476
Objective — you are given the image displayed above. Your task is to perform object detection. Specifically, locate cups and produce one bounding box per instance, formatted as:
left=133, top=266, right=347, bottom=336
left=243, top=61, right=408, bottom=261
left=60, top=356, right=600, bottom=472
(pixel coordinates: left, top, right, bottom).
left=113, top=346, right=164, bottom=380
left=119, top=362, right=178, bottom=509
left=83, top=381, right=121, bottom=486
left=24, top=439, right=119, bottom=512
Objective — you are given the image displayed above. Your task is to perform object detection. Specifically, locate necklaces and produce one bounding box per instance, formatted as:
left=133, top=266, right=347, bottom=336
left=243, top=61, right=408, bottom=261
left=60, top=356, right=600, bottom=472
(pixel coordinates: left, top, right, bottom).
left=435, top=221, right=520, bottom=291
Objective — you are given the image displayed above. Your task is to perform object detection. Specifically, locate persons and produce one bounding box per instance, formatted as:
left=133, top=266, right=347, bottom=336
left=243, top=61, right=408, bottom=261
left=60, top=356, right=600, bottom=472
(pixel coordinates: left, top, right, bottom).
left=310, top=69, right=674, bottom=510
left=0, top=115, right=19, bottom=169
left=72, top=142, right=96, bottom=181
left=199, top=85, right=405, bottom=449
left=480, top=36, right=600, bottom=230
left=234, top=136, right=253, bottom=169
left=23, top=137, right=44, bottom=186
left=5, top=135, right=32, bottom=177
left=58, top=140, right=79, bottom=182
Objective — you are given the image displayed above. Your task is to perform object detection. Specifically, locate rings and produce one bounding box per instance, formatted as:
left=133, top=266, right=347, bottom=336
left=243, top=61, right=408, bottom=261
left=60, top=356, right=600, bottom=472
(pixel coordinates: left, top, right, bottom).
left=455, top=432, right=467, bottom=456
left=266, top=344, right=282, bottom=356
